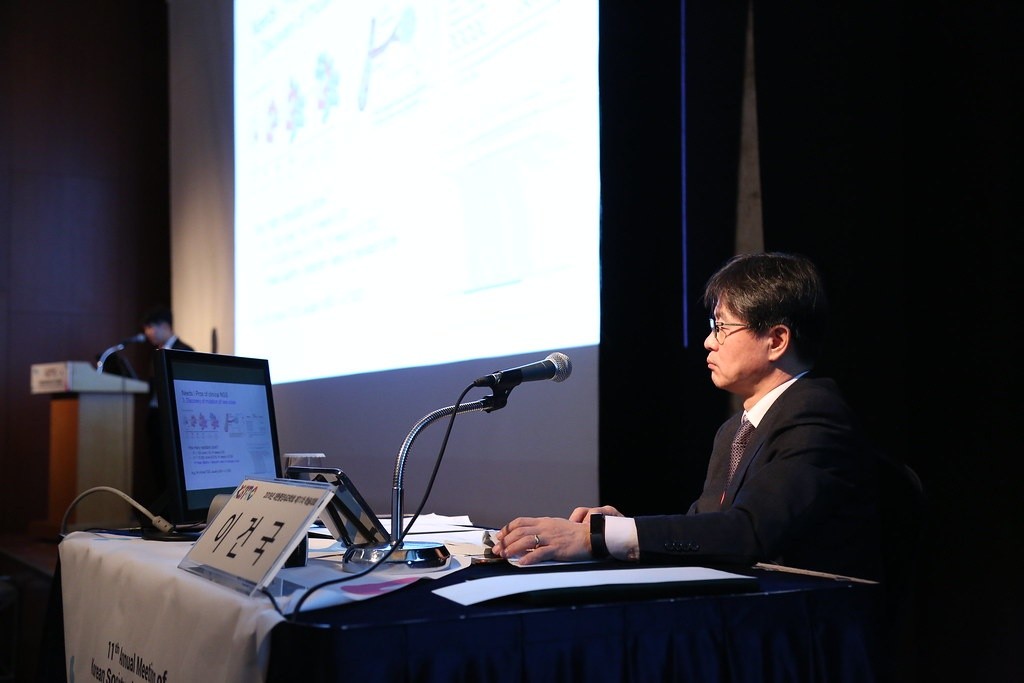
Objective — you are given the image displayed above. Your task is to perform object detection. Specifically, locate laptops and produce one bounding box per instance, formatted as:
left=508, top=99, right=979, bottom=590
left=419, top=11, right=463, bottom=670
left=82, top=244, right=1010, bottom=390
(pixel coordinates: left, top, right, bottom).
left=95, top=352, right=139, bottom=380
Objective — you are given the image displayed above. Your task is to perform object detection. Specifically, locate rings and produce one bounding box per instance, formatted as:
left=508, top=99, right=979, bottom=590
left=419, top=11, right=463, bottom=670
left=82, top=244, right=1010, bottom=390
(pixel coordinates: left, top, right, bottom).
left=534, top=533, right=540, bottom=549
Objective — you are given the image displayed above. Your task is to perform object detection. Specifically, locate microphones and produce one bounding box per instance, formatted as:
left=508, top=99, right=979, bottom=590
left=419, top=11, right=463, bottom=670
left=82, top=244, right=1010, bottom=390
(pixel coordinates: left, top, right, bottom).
left=474, top=353, right=572, bottom=386
left=121, top=333, right=146, bottom=344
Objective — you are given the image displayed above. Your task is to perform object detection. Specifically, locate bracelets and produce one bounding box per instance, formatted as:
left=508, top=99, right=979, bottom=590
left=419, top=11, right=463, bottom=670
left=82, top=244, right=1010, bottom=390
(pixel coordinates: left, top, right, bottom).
left=588, top=512, right=614, bottom=560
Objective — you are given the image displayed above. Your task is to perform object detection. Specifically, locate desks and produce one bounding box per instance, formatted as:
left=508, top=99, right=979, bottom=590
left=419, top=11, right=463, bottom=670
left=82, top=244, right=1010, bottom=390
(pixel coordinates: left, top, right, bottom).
left=57, top=513, right=893, bottom=683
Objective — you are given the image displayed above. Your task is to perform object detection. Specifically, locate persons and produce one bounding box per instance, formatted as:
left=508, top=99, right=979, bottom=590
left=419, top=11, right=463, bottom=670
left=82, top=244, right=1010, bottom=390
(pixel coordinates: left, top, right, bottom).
left=492, top=252, right=871, bottom=579
left=142, top=305, right=194, bottom=351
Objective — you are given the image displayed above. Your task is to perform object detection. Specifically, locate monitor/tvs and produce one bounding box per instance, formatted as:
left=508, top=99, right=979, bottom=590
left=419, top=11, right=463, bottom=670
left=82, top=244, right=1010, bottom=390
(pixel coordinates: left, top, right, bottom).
left=151, top=349, right=282, bottom=524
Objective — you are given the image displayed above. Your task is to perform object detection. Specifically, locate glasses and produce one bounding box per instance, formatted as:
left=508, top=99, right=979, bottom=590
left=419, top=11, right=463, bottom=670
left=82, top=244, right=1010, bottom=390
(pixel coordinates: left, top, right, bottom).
left=710, top=318, right=756, bottom=339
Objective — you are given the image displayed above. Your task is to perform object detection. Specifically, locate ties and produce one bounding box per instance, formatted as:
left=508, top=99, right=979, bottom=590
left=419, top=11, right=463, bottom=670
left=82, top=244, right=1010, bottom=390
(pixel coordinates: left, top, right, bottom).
left=720, top=420, right=756, bottom=504
